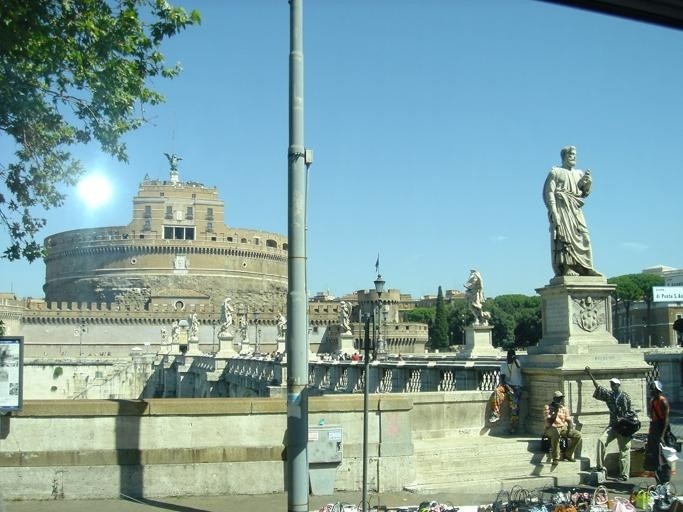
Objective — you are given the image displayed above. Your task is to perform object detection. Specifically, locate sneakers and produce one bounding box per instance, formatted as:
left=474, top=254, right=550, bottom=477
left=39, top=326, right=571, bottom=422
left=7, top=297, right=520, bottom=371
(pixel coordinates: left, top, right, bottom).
left=552, top=460, right=558, bottom=464
left=489, top=415, right=501, bottom=423
left=593, top=465, right=603, bottom=471
left=619, top=474, right=627, bottom=480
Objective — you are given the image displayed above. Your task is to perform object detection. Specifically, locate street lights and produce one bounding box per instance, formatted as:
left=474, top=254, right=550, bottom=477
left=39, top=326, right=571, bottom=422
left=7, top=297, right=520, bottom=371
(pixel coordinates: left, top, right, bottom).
left=253, top=309, right=261, bottom=357
left=374, top=274, right=388, bottom=362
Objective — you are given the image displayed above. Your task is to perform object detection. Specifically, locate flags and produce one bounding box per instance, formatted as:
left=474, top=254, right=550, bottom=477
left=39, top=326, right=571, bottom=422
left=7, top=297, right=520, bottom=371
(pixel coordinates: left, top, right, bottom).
left=374, top=253, right=378, bottom=273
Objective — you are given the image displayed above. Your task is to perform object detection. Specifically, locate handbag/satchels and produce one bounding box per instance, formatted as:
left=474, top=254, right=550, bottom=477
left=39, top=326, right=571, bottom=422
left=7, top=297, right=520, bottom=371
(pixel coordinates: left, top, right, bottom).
left=478, top=481, right=683, bottom=512
left=616, top=417, right=641, bottom=437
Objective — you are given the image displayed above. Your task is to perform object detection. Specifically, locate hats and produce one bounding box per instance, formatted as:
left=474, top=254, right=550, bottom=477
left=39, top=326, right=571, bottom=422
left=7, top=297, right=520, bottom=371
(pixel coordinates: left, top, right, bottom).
left=609, top=378, right=620, bottom=384
left=654, top=380, right=663, bottom=392
left=552, top=390, right=565, bottom=399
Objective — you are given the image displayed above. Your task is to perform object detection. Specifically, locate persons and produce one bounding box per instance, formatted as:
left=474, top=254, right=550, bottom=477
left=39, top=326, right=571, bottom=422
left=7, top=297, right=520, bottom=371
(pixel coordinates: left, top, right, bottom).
left=335, top=301, right=351, bottom=333
left=542, top=145, right=605, bottom=277
left=158, top=296, right=287, bottom=344
left=488, top=348, right=523, bottom=432
left=639, top=379, right=677, bottom=478
left=462, top=268, right=488, bottom=326
left=543, top=389, right=582, bottom=465
left=241, top=348, right=406, bottom=378
left=672, top=313, right=683, bottom=348
left=583, top=365, right=639, bottom=482
